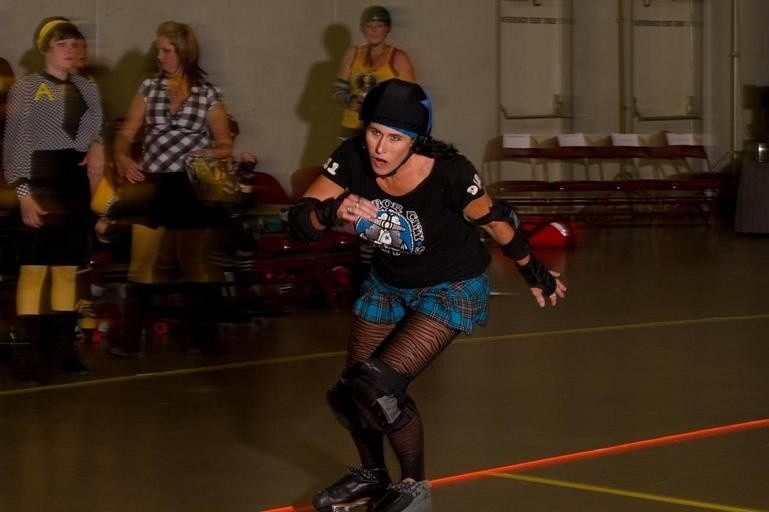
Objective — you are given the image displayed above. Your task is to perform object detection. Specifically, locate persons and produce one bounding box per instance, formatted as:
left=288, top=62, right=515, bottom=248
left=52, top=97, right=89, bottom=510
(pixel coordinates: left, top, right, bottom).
left=2, top=9, right=115, bottom=379
left=287, top=77, right=573, bottom=512
left=111, top=16, right=234, bottom=357
left=329, top=2, right=418, bottom=149
left=1, top=58, right=262, bottom=346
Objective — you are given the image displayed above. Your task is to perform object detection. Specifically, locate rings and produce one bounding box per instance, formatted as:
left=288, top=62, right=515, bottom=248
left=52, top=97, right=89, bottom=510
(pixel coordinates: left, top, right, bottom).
left=346, top=206, right=354, bottom=213
left=355, top=202, right=360, bottom=208
left=358, top=196, right=362, bottom=202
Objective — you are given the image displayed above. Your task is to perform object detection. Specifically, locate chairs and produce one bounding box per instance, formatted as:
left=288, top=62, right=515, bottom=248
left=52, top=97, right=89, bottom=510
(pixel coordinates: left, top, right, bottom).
left=253, top=171, right=295, bottom=316
left=287, top=167, right=362, bottom=313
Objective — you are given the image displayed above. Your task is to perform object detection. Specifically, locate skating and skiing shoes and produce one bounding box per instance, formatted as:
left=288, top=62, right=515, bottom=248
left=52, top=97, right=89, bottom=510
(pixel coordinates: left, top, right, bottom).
left=75, top=299, right=101, bottom=343
left=313, top=464, right=432, bottom=512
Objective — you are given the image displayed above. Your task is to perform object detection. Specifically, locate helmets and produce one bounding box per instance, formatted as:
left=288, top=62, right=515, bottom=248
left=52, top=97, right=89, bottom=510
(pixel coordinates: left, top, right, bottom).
left=359, top=78, right=432, bottom=136
left=359, top=6, right=389, bottom=22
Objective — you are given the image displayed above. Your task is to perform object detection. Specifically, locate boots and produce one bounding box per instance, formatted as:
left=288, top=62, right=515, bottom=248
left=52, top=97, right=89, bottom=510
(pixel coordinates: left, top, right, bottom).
left=15, top=310, right=88, bottom=383
left=185, top=282, right=219, bottom=356
left=109, top=281, right=151, bottom=355
